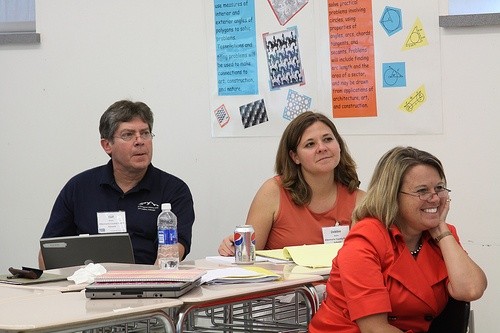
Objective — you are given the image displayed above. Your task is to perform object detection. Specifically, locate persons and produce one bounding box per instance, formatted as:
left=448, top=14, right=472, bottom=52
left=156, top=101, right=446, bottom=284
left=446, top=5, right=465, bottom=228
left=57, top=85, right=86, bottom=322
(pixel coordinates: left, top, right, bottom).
left=305, top=147, right=487, bottom=333
left=218, top=112, right=367, bottom=256
left=38, top=100, right=195, bottom=271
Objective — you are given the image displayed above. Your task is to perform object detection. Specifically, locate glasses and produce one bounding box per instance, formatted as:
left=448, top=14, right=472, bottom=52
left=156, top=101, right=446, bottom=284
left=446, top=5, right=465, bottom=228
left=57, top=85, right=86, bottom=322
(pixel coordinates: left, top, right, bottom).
left=113, top=131, right=155, bottom=141
left=399, top=188, right=452, bottom=202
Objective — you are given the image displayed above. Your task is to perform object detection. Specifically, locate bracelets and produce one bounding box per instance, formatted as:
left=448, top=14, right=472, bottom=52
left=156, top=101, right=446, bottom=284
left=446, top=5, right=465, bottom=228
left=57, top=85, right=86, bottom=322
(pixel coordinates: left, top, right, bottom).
left=434, top=231, right=453, bottom=243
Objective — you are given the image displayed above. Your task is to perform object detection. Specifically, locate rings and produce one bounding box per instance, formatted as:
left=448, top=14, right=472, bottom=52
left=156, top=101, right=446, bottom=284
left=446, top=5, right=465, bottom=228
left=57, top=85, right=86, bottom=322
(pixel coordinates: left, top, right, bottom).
left=446, top=199, right=451, bottom=202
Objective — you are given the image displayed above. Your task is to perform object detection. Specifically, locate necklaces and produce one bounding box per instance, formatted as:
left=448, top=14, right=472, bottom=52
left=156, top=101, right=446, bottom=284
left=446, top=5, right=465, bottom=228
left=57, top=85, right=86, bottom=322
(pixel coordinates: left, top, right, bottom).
left=410, top=244, right=423, bottom=255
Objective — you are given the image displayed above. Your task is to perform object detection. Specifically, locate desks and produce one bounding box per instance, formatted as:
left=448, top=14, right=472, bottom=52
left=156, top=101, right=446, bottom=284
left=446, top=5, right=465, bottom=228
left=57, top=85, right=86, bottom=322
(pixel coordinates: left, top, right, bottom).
left=0, top=257, right=336, bottom=333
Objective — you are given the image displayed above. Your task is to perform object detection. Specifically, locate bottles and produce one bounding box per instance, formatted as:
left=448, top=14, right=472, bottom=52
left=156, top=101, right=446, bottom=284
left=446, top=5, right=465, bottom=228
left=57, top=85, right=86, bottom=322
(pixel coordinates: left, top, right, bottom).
left=156, top=203, right=180, bottom=269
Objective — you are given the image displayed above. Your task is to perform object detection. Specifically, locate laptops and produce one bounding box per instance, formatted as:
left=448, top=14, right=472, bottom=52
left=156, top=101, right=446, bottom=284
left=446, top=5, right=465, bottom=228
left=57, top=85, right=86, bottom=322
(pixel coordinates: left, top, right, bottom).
left=84, top=278, right=201, bottom=298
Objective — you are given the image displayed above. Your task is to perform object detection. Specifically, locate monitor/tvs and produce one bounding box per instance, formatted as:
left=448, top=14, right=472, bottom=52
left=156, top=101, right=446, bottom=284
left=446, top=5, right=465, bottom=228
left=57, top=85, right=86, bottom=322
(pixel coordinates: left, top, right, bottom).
left=40, top=234, right=135, bottom=270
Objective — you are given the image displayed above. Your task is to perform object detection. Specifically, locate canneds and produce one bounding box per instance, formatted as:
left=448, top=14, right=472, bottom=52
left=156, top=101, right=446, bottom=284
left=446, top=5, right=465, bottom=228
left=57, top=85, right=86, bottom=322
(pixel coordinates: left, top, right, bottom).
left=234, top=225, right=256, bottom=265
